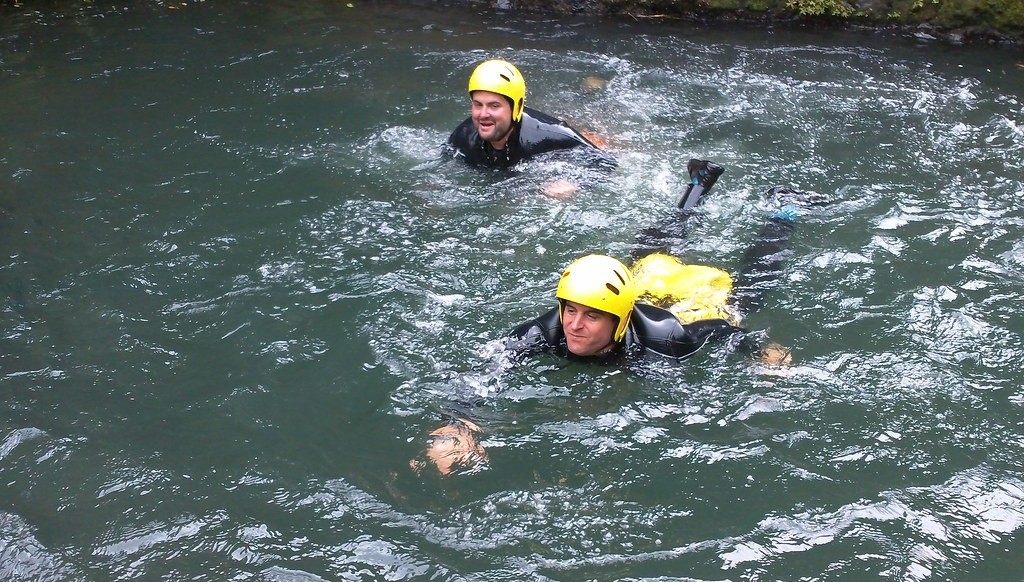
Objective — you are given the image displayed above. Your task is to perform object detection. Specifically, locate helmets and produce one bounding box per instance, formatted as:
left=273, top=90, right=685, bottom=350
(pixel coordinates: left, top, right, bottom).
left=468, top=60, right=525, bottom=124
left=557, top=255, right=635, bottom=342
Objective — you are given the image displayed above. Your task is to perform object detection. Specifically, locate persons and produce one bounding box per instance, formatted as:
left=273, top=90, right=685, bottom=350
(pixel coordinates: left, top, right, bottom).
left=512, top=160, right=725, bottom=360
left=447, top=59, right=589, bottom=163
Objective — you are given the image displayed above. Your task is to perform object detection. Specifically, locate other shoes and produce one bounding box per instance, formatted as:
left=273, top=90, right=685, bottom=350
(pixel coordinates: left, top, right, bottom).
left=687, top=160, right=724, bottom=195
left=766, top=186, right=834, bottom=210
